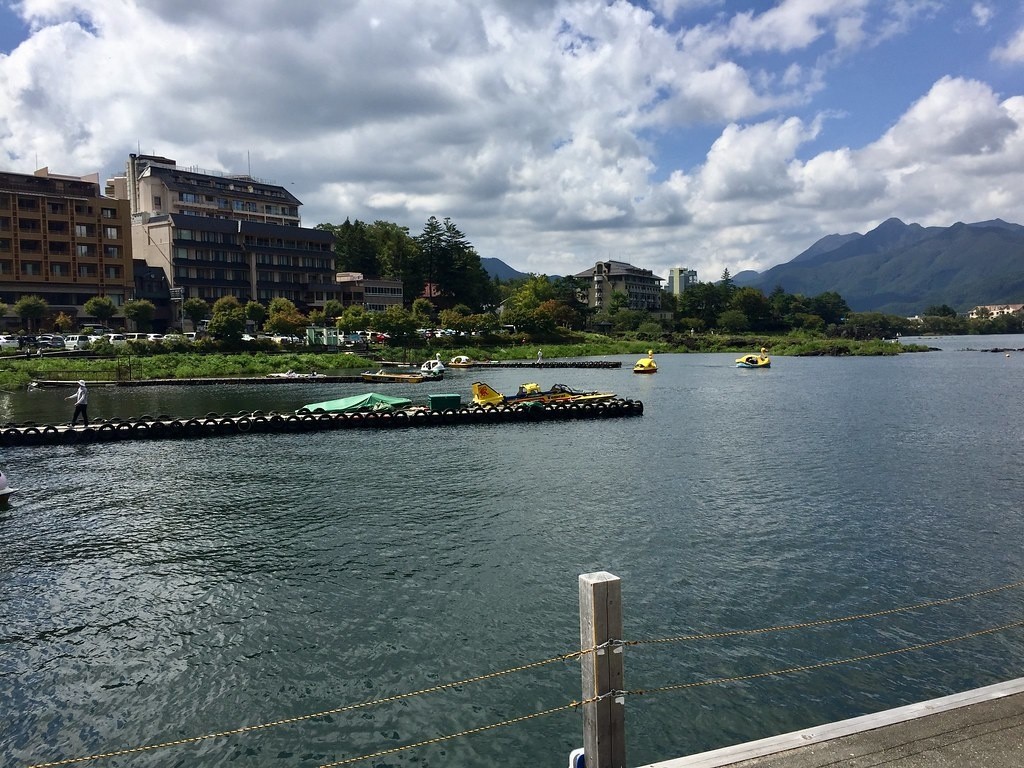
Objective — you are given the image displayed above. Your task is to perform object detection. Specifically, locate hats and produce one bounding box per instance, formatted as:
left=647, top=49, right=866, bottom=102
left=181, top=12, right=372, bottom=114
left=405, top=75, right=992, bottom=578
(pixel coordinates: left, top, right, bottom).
left=77, top=380, right=86, bottom=387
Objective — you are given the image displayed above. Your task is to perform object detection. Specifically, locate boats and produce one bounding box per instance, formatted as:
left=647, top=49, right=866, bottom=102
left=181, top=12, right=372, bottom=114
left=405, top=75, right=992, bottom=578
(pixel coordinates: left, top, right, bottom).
left=735, top=347, right=772, bottom=370
left=471, top=381, right=617, bottom=407
left=418, top=352, right=447, bottom=381
left=361, top=370, right=424, bottom=384
left=632, top=350, right=660, bottom=373
left=448, top=356, right=474, bottom=368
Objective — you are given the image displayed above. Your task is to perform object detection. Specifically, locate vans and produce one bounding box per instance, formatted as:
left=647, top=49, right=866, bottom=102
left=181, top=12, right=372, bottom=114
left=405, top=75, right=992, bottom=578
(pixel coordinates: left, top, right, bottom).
left=102, top=334, right=126, bottom=347
left=333, top=331, right=393, bottom=344
left=164, top=334, right=182, bottom=343
left=64, top=335, right=90, bottom=350
left=121, top=332, right=148, bottom=344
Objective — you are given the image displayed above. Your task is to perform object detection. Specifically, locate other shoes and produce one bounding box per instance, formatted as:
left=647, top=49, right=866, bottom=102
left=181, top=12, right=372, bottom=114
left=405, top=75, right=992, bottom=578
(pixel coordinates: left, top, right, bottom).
left=67, top=423, right=74, bottom=429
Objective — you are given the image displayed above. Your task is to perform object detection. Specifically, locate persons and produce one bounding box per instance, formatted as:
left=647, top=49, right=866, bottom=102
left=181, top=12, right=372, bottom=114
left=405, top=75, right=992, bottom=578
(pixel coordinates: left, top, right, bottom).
left=691, top=328, right=694, bottom=337
left=536, top=349, right=543, bottom=363
left=64, top=379, right=90, bottom=427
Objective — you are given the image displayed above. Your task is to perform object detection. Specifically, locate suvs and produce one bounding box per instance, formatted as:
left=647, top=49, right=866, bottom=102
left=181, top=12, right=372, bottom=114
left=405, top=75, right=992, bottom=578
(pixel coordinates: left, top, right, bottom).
left=147, top=334, right=164, bottom=346
left=183, top=332, right=198, bottom=342
left=36, top=335, right=66, bottom=350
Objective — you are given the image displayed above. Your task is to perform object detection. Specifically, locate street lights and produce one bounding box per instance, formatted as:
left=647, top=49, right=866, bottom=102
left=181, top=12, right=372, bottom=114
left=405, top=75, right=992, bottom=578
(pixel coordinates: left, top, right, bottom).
left=415, top=325, right=517, bottom=337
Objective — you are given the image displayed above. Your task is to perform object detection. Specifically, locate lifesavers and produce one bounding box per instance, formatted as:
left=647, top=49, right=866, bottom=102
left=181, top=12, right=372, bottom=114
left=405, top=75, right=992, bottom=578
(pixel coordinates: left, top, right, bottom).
left=41, top=427, right=59, bottom=444
left=99, top=396, right=641, bottom=444
left=22, top=427, right=41, bottom=446
left=79, top=428, right=97, bottom=445
left=514, top=361, right=610, bottom=368
left=61, top=429, right=79, bottom=446
left=3, top=428, right=22, bottom=447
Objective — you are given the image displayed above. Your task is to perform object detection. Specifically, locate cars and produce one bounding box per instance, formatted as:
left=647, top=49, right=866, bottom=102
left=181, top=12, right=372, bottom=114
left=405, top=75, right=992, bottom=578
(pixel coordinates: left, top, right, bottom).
left=87, top=336, right=105, bottom=346
left=240, top=333, right=256, bottom=342
left=256, top=331, right=300, bottom=344
left=15, top=335, right=38, bottom=350
left=0, top=335, right=20, bottom=350
